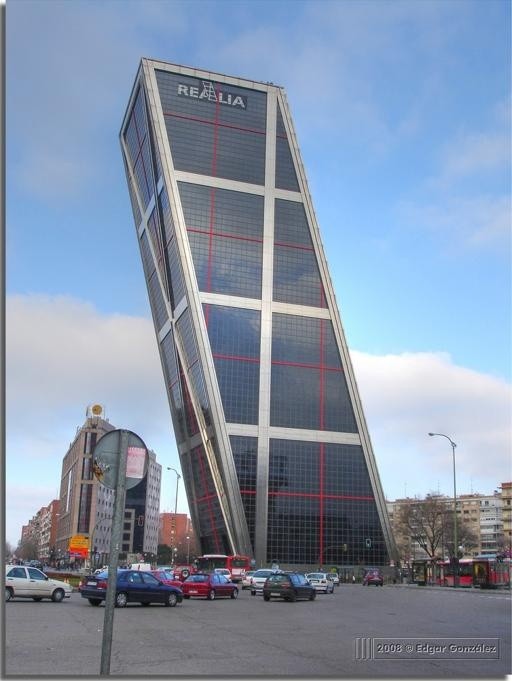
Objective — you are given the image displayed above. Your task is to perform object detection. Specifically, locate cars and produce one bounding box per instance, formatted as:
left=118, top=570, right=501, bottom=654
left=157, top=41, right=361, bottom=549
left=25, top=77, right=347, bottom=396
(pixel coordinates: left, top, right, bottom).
left=262, top=571, right=317, bottom=602
left=402, top=567, right=411, bottom=576
left=361, top=567, right=384, bottom=586
left=327, top=573, right=340, bottom=587
left=78, top=570, right=184, bottom=608
left=12, top=559, right=45, bottom=572
left=94, top=561, right=232, bottom=589
left=181, top=573, right=239, bottom=601
left=241, top=572, right=256, bottom=591
left=249, top=569, right=283, bottom=596
left=5, top=564, right=73, bottom=605
left=305, top=573, right=335, bottom=595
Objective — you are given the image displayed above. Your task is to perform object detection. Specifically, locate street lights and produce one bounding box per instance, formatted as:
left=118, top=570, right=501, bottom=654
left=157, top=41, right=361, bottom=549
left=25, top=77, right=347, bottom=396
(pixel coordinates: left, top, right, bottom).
left=166, top=466, right=181, bottom=563
left=186, top=536, right=190, bottom=562
left=427, top=432, right=460, bottom=590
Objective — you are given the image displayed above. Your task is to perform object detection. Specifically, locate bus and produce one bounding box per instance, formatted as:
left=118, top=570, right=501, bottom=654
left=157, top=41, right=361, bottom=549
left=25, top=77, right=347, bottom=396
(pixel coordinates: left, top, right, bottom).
left=433, top=556, right=509, bottom=590
left=195, top=554, right=252, bottom=584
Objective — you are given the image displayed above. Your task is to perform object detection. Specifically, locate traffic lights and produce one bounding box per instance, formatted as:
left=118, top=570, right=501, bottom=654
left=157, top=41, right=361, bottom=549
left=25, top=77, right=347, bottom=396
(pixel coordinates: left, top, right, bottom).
left=139, top=516, right=143, bottom=525
left=367, top=540, right=371, bottom=547
